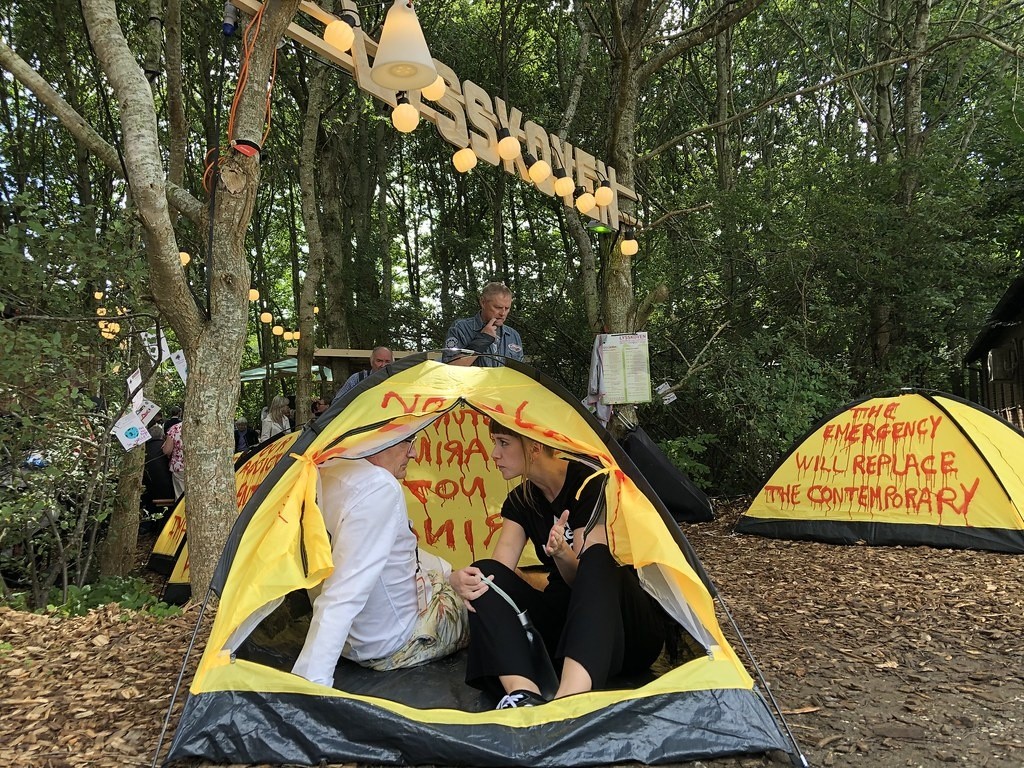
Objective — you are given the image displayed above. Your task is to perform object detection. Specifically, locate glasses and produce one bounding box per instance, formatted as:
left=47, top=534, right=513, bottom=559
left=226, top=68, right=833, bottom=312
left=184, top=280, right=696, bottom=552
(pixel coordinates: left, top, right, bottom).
left=404, top=436, right=419, bottom=453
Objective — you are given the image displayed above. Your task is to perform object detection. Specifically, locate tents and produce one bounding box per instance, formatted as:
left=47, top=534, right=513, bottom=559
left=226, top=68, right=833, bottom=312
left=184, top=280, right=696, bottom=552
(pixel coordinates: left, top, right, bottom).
left=158, top=423, right=308, bottom=610
left=732, top=386, right=1024, bottom=553
left=146, top=442, right=260, bottom=579
left=147, top=346, right=810, bottom=768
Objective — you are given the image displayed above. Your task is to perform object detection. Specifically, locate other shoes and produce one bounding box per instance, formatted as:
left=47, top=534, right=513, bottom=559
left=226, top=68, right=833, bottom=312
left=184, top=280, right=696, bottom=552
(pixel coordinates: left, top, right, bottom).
left=493, top=690, right=548, bottom=710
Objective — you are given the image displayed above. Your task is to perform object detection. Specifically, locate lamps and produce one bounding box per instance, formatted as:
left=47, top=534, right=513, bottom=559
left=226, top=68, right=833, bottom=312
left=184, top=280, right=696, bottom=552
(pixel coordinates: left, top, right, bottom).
left=572, top=186, right=597, bottom=213
left=585, top=219, right=616, bottom=234
left=322, top=8, right=363, bottom=53
left=370, top=0, right=438, bottom=92
left=525, top=154, right=552, bottom=185
left=497, top=127, right=521, bottom=161
left=620, top=230, right=639, bottom=256
left=95, top=246, right=321, bottom=375
left=594, top=180, right=613, bottom=207
left=421, top=74, right=446, bottom=102
left=390, top=90, right=420, bottom=132
left=553, top=167, right=576, bottom=197
left=452, top=145, right=478, bottom=173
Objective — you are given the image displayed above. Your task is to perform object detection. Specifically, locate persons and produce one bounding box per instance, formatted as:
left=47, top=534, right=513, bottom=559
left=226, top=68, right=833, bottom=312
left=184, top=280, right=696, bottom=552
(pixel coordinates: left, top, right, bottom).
left=284, top=431, right=550, bottom=691
left=137, top=395, right=334, bottom=535
left=330, top=346, right=396, bottom=406
left=439, top=281, right=524, bottom=368
left=448, top=417, right=679, bottom=711
